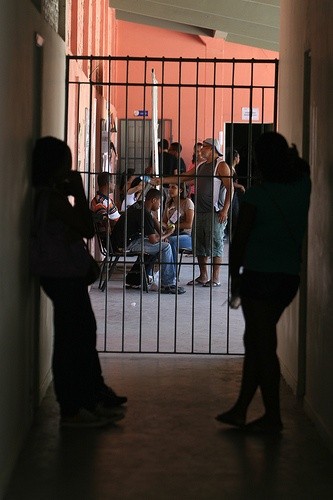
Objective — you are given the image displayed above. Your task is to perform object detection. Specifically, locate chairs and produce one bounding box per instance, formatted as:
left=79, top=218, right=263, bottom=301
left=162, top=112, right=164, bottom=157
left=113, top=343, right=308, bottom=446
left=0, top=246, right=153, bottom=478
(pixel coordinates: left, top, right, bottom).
left=178, top=249, right=193, bottom=281
left=95, top=220, right=150, bottom=294
